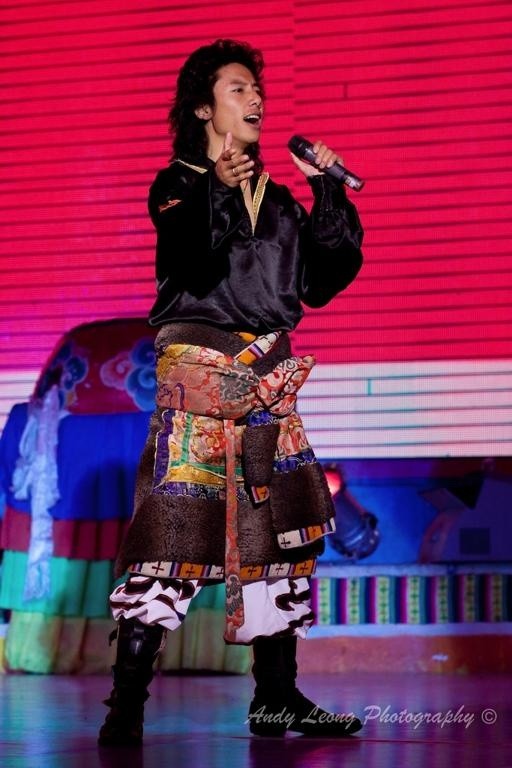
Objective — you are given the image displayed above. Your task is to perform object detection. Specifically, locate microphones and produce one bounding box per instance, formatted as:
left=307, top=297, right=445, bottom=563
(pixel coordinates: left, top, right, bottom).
left=288, top=135, right=363, bottom=192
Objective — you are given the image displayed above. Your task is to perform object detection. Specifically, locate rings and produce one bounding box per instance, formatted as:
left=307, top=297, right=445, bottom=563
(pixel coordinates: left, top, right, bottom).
left=232, top=168, right=237, bottom=175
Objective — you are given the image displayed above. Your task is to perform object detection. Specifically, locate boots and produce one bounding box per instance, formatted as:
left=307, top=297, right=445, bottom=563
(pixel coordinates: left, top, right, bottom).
left=247, top=635, right=363, bottom=737
left=97, top=614, right=165, bottom=756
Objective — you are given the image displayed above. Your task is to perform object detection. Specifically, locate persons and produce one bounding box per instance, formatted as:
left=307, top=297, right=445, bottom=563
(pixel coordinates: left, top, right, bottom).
left=98, top=38, right=364, bottom=748
